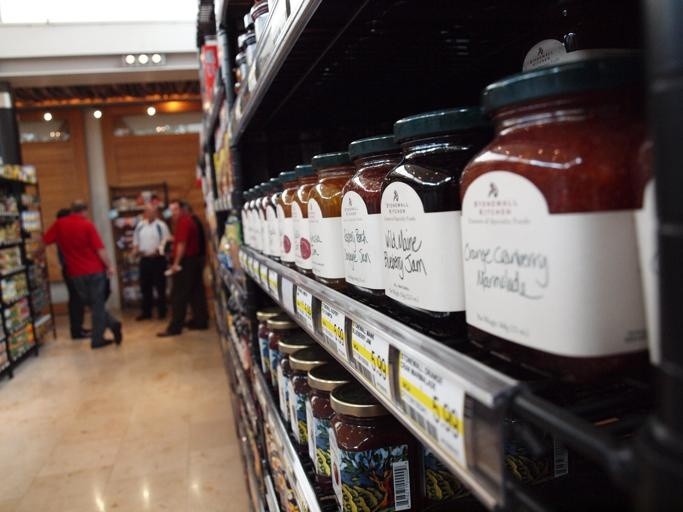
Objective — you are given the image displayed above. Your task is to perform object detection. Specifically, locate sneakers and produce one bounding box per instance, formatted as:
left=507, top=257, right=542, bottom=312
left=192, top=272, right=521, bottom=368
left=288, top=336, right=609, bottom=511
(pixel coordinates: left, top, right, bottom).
left=136, top=314, right=152, bottom=320
left=79, top=329, right=92, bottom=338
left=112, top=321, right=122, bottom=344
left=92, top=340, right=112, bottom=348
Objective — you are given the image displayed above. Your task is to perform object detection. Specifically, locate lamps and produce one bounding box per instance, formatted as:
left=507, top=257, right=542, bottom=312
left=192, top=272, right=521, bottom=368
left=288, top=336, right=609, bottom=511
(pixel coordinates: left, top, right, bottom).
left=120, top=53, right=166, bottom=66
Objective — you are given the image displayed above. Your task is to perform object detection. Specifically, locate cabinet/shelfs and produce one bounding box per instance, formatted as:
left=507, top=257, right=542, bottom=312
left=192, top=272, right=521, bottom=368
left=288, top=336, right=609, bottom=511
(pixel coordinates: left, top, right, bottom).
left=110, top=181, right=173, bottom=309
left=195, top=0, right=682, bottom=512
left=0, top=174, right=57, bottom=377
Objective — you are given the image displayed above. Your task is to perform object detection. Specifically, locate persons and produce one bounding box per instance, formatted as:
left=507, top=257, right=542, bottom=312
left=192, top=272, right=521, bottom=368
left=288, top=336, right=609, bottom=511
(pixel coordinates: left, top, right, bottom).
left=41, top=198, right=210, bottom=348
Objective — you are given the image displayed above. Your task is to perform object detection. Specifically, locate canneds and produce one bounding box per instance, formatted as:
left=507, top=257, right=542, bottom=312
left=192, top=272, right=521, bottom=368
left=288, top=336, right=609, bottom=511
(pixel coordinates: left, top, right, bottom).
left=195, top=0, right=683, bottom=428
left=214, top=262, right=484, bottom=511
